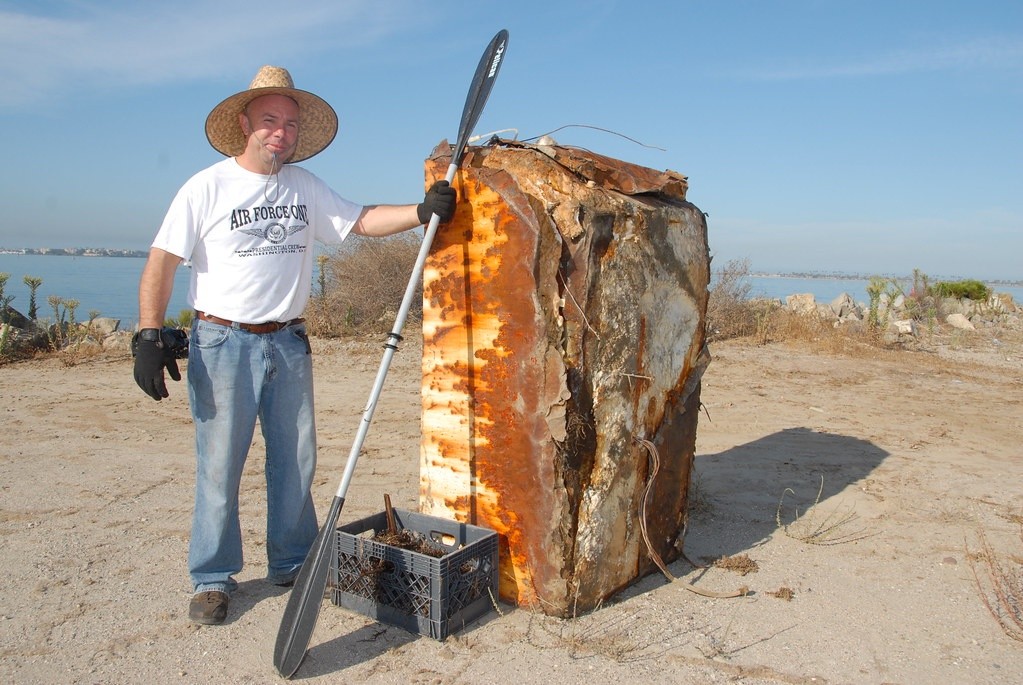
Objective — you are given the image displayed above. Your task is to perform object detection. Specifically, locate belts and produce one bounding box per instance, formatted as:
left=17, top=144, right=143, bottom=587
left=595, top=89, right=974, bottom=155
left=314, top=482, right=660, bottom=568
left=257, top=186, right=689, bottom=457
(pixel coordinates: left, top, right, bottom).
left=195, top=310, right=306, bottom=333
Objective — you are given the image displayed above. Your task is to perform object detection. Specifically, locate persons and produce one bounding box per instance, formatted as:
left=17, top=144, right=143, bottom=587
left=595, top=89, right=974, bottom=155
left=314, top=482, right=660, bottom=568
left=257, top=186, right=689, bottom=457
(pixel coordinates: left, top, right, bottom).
left=133, top=65, right=457, bottom=626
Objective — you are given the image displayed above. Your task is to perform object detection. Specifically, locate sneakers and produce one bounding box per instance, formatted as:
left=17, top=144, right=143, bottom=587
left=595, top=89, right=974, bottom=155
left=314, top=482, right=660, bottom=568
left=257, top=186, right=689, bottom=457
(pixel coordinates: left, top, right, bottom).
left=189, top=591, right=229, bottom=624
left=280, top=579, right=334, bottom=599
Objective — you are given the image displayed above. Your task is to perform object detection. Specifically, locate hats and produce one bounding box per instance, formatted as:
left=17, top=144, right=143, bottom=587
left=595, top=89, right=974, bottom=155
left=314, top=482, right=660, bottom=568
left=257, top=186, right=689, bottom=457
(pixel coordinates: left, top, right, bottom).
left=205, top=65, right=338, bottom=165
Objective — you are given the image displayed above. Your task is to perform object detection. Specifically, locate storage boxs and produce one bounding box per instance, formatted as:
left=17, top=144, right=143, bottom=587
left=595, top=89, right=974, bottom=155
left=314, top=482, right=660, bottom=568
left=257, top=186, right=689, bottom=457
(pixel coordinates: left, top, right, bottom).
left=329, top=507, right=498, bottom=642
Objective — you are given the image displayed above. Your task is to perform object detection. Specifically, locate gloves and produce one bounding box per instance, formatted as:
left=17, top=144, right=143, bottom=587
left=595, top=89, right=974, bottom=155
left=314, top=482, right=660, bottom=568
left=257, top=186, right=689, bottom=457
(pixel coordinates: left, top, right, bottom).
left=133, top=327, right=181, bottom=400
left=417, top=179, right=457, bottom=224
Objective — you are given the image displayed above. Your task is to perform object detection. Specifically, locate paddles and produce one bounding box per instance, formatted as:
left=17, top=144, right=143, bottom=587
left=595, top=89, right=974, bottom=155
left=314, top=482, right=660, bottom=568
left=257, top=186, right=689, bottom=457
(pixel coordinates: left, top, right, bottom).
left=271, top=26, right=512, bottom=681
